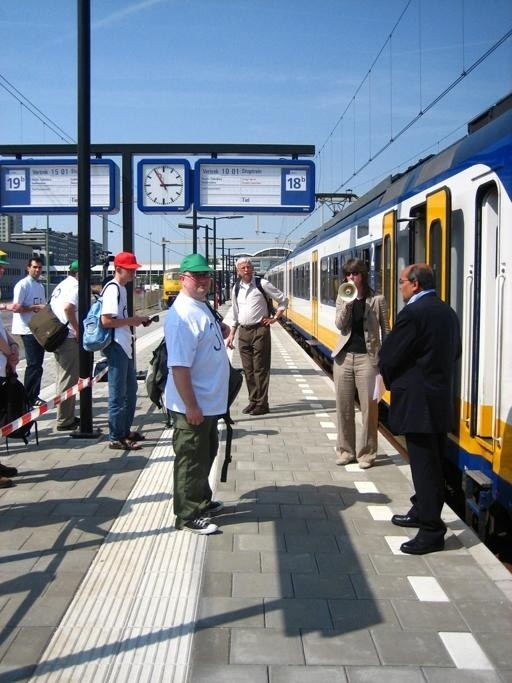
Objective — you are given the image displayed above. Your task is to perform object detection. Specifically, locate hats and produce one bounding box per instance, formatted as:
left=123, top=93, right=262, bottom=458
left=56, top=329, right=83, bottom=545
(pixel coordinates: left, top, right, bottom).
left=69, top=260, right=78, bottom=274
left=179, top=253, right=215, bottom=277
left=113, top=251, right=142, bottom=271
left=0, top=250, right=10, bottom=265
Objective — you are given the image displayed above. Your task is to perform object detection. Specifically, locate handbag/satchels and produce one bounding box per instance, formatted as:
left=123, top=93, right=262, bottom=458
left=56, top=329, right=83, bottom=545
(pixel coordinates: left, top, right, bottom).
left=27, top=301, right=68, bottom=352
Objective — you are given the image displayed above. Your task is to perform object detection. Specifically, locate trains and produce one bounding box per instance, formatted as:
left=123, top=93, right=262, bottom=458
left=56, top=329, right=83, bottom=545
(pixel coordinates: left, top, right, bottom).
left=162, top=266, right=184, bottom=309
left=260, top=92, right=511, bottom=563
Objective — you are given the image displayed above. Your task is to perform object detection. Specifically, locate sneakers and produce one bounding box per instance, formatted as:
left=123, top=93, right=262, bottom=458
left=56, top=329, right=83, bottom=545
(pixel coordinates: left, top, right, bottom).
left=180, top=517, right=219, bottom=534
left=0, top=463, right=18, bottom=477
left=205, top=498, right=226, bottom=512
left=0, top=477, right=13, bottom=489
left=56, top=415, right=82, bottom=431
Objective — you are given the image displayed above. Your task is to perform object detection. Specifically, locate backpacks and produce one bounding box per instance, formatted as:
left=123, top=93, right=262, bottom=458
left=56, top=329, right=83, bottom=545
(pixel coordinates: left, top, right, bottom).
left=0, top=367, right=38, bottom=443
left=81, top=280, right=122, bottom=353
left=145, top=338, right=243, bottom=421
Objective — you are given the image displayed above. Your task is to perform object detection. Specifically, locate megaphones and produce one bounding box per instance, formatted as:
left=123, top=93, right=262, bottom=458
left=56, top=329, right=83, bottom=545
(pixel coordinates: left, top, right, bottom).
left=338, top=281, right=358, bottom=303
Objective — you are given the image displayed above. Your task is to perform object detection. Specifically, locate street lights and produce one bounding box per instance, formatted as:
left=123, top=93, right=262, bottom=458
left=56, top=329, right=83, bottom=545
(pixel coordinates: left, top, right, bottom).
left=148, top=230, right=154, bottom=291
left=177, top=221, right=213, bottom=303
left=203, top=234, right=246, bottom=306
left=186, top=215, right=246, bottom=318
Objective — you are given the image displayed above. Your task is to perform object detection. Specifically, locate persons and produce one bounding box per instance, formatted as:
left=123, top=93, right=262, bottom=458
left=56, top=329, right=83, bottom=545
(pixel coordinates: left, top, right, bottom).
left=163, top=254, right=231, bottom=535
left=47, top=259, right=82, bottom=431
left=96, top=251, right=149, bottom=451
left=0, top=248, right=45, bottom=489
left=329, top=259, right=390, bottom=470
left=225, top=257, right=289, bottom=416
left=378, top=261, right=462, bottom=555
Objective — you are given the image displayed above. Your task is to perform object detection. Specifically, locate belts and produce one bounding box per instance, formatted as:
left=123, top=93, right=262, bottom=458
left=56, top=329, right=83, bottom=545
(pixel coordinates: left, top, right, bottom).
left=240, top=324, right=262, bottom=330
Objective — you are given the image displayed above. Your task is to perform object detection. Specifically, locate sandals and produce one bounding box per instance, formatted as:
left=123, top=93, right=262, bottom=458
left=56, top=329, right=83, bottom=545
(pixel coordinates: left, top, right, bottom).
left=108, top=429, right=146, bottom=450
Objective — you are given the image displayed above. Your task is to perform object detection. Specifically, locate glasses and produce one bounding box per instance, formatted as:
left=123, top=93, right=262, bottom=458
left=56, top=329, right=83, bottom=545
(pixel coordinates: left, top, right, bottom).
left=344, top=271, right=361, bottom=277
left=183, top=272, right=212, bottom=280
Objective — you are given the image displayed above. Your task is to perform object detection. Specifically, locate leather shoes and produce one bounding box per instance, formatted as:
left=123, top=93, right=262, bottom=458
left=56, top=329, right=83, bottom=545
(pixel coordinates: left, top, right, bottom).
left=400, top=534, right=444, bottom=556
left=392, top=514, right=419, bottom=529
left=358, top=461, right=376, bottom=469
left=242, top=402, right=269, bottom=415
left=336, top=455, right=356, bottom=466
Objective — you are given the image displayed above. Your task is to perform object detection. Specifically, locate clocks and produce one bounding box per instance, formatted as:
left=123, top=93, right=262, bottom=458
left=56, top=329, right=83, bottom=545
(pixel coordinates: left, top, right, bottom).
left=137, top=157, right=192, bottom=214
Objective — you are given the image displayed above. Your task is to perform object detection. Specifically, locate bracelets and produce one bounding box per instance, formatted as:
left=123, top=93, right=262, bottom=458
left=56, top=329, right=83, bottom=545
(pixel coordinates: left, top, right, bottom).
left=273, top=315, right=279, bottom=321
